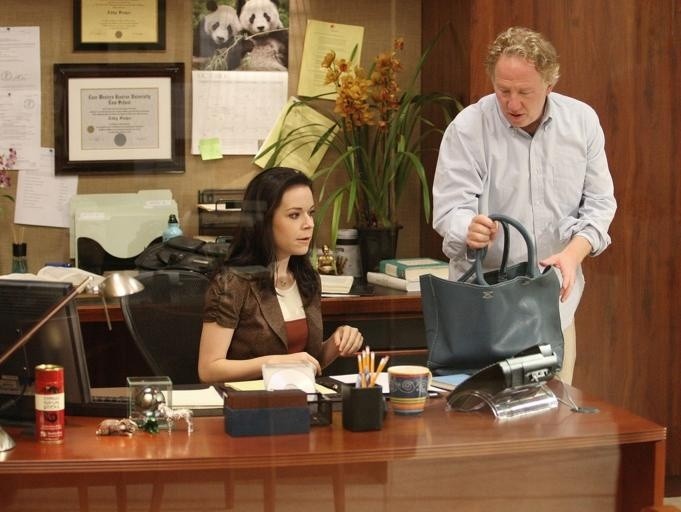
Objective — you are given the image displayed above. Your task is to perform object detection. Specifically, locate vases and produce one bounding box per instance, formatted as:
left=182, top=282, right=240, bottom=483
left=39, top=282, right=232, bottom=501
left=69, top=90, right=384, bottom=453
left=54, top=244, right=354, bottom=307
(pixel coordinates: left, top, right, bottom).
left=353, top=224, right=405, bottom=286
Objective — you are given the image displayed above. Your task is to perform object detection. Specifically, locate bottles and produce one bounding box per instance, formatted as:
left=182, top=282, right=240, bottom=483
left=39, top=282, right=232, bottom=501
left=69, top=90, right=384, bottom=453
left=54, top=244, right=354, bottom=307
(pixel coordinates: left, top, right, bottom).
left=335, top=226, right=362, bottom=286
left=10, top=242, right=28, bottom=273
left=161, top=214, right=183, bottom=242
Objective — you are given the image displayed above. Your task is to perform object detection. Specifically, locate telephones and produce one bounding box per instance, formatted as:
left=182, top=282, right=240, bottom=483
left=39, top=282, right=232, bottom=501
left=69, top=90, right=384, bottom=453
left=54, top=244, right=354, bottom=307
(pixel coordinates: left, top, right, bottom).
left=446, top=343, right=558, bottom=412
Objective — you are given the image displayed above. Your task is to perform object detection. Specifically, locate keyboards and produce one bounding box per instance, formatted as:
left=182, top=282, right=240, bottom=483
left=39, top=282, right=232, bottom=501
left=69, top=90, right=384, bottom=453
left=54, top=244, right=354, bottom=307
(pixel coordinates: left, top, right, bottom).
left=81, top=395, right=138, bottom=418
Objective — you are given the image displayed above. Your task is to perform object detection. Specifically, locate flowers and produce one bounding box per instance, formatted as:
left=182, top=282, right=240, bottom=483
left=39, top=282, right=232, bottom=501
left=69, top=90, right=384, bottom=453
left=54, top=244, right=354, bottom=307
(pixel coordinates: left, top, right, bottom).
left=252, top=37, right=467, bottom=274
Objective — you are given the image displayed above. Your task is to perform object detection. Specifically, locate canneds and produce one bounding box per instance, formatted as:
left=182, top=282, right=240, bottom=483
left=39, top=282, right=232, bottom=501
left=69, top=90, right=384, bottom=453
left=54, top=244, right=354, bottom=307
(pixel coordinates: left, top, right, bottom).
left=33, top=362, right=67, bottom=444
left=335, top=228, right=364, bottom=279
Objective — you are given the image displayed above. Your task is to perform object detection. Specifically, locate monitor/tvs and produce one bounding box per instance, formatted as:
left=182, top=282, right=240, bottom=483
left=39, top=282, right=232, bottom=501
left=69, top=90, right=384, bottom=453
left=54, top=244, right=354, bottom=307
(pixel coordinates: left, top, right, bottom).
left=0, top=279, right=91, bottom=404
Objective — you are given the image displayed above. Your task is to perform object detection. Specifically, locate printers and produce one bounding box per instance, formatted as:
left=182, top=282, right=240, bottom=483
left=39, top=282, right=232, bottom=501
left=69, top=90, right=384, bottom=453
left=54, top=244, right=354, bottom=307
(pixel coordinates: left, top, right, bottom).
left=197, top=187, right=245, bottom=236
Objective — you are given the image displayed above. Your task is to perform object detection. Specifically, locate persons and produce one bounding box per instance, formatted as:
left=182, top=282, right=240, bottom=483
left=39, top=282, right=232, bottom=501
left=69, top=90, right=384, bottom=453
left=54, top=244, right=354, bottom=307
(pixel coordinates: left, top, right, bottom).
left=197, top=166, right=365, bottom=382
left=430, top=24, right=619, bottom=387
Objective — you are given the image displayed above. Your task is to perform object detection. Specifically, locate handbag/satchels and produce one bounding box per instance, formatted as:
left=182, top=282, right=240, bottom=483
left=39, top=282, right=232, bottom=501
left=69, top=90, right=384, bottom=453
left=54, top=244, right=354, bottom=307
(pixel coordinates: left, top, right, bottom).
left=420, top=214, right=567, bottom=375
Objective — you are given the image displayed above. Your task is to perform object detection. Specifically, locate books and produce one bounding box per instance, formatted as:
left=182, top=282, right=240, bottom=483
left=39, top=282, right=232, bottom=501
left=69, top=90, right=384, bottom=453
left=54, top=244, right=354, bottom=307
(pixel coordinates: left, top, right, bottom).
left=317, top=274, right=355, bottom=294
left=366, top=269, right=422, bottom=293
left=328, top=370, right=391, bottom=395
left=320, top=284, right=362, bottom=299
left=142, top=385, right=225, bottom=410
left=430, top=372, right=473, bottom=391
left=379, top=257, right=449, bottom=284
left=0, top=263, right=107, bottom=289
left=222, top=377, right=337, bottom=402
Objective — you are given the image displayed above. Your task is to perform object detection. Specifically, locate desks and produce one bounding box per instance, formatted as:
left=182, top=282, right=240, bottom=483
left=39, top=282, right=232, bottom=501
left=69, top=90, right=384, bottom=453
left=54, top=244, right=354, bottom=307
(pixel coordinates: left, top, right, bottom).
left=0, top=371, right=670, bottom=509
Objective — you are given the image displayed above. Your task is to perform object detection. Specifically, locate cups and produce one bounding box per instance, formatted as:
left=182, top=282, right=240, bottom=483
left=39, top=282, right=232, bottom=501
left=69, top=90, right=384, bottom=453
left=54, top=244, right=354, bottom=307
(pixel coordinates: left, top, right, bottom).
left=339, top=382, right=384, bottom=431
left=387, top=365, right=433, bottom=415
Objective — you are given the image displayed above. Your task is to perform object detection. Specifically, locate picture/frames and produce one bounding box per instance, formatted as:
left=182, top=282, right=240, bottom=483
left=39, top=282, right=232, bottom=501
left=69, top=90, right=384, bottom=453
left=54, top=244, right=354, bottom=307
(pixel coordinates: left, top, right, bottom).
left=53, top=1, right=186, bottom=175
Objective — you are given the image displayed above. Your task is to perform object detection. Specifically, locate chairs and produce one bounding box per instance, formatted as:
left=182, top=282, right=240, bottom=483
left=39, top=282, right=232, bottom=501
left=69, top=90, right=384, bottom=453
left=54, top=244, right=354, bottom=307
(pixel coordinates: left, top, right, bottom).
left=119, top=268, right=213, bottom=384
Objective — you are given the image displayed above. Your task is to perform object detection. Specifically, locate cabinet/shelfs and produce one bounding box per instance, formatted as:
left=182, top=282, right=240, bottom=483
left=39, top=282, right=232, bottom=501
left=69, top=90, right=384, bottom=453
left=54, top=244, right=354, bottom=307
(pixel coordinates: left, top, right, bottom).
left=421, top=3, right=681, bottom=496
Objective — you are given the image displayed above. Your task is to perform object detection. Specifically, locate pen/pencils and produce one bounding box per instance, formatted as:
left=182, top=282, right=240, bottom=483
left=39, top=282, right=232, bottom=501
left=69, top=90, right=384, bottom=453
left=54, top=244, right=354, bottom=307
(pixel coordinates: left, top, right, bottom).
left=10, top=223, right=24, bottom=245
left=357, top=345, right=390, bottom=389
